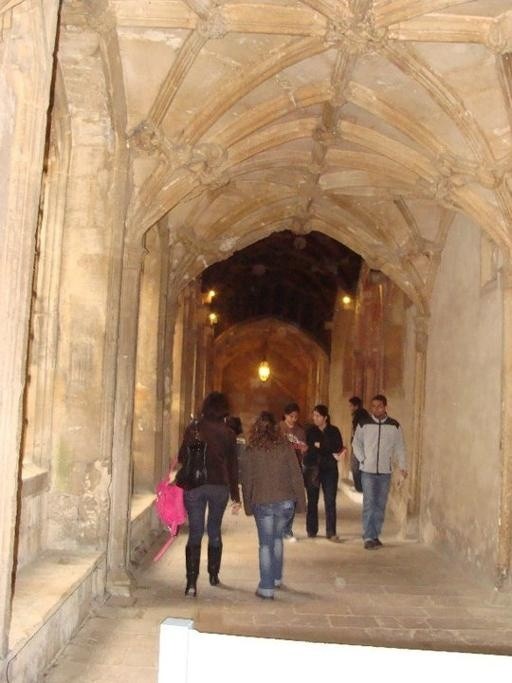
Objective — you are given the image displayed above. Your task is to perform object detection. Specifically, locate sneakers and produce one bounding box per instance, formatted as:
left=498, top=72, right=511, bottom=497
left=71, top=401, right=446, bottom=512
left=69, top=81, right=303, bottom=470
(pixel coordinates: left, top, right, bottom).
left=361, top=538, right=382, bottom=549
left=326, top=534, right=339, bottom=542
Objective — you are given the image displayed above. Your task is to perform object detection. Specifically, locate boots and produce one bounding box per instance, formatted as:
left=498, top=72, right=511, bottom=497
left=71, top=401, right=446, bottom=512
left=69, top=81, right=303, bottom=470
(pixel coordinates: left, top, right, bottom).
left=208, top=543, right=222, bottom=587
left=185, top=544, right=200, bottom=596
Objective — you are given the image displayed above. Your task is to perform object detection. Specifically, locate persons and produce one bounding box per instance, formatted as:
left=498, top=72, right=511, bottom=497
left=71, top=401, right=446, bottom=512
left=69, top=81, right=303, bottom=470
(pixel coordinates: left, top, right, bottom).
left=303, top=405, right=342, bottom=543
left=351, top=394, right=409, bottom=551
left=222, top=416, right=246, bottom=450
left=242, top=412, right=306, bottom=599
left=348, top=396, right=369, bottom=493
left=178, top=391, right=241, bottom=595
left=278, top=404, right=308, bottom=539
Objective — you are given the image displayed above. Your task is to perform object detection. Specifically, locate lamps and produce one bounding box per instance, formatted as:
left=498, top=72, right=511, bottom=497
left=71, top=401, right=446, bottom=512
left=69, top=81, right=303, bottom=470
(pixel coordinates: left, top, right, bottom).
left=255, top=355, right=275, bottom=383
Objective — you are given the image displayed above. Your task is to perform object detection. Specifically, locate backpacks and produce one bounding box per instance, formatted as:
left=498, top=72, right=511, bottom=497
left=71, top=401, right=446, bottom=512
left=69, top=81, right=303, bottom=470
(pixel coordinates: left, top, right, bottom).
left=154, top=475, right=188, bottom=536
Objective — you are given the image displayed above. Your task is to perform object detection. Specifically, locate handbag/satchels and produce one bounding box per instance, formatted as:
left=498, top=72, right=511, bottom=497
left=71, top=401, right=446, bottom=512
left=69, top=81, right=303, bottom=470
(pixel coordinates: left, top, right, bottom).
left=174, top=418, right=208, bottom=491
left=302, top=447, right=321, bottom=489
